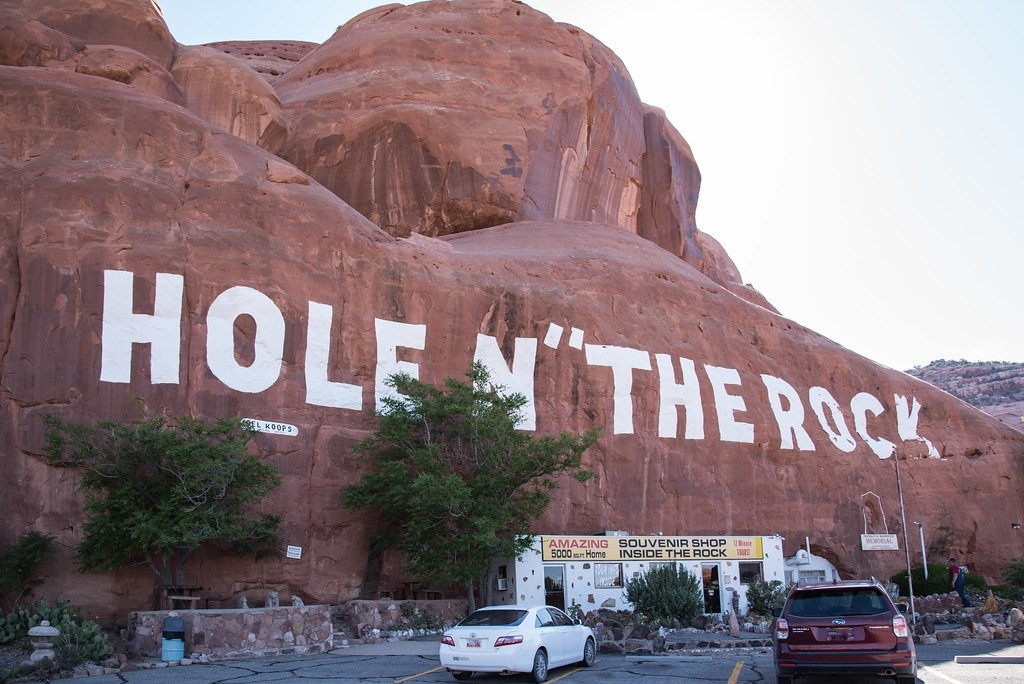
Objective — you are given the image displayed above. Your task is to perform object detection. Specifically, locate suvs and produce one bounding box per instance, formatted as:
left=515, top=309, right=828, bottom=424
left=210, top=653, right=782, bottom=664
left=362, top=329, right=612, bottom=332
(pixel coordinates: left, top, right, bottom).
left=773, top=573, right=921, bottom=684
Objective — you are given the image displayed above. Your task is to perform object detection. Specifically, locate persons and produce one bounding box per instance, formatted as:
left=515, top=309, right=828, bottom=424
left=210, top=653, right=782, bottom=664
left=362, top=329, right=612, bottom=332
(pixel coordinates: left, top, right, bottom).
left=948, top=557, right=974, bottom=607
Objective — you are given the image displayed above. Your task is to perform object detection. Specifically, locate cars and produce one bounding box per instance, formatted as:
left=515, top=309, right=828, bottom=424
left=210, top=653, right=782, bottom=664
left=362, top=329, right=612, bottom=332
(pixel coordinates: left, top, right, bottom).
left=438, top=605, right=596, bottom=684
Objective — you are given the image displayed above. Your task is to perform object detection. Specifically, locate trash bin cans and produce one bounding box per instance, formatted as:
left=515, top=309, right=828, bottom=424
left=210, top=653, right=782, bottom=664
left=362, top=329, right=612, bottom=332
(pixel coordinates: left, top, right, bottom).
left=161, top=617, right=186, bottom=663
